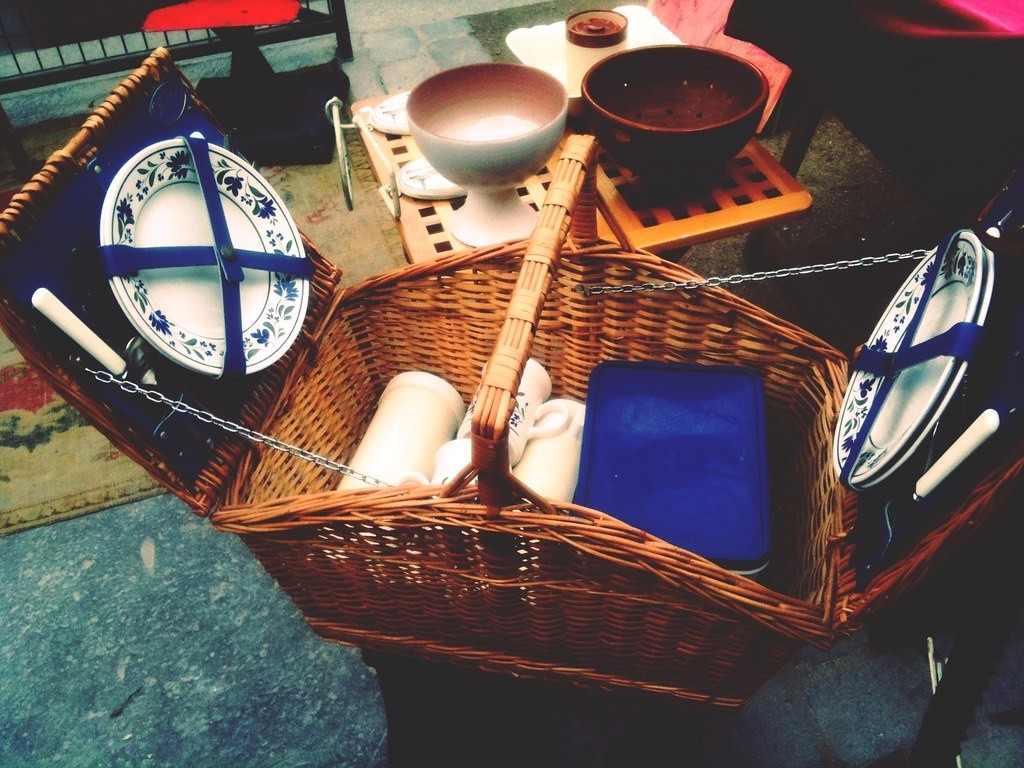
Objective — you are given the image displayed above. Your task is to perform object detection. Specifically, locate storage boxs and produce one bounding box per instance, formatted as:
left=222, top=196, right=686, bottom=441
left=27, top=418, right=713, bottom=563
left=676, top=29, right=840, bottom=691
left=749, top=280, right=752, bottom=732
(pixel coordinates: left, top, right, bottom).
left=570, top=364, right=776, bottom=575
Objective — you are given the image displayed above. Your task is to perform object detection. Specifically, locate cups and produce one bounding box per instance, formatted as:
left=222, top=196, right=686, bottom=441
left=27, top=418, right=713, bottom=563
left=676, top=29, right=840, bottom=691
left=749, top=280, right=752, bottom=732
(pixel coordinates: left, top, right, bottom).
left=337, top=359, right=587, bottom=505
left=565, top=9, right=628, bottom=99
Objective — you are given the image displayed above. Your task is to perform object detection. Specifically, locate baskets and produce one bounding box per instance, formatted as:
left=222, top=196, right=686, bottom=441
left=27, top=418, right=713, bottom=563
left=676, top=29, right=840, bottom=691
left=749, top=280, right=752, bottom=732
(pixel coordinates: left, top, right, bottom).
left=2, top=46, right=1022, bottom=714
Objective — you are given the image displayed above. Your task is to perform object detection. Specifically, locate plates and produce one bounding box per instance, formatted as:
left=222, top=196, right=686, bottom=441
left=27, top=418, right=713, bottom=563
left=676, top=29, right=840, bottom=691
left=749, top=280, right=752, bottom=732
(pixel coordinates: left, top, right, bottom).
left=100, top=138, right=309, bottom=375
left=833, top=232, right=994, bottom=487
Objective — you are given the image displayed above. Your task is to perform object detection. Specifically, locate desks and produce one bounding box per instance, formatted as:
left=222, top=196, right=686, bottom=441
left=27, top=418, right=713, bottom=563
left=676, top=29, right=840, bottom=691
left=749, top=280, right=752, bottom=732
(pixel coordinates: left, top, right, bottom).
left=350, top=93, right=814, bottom=263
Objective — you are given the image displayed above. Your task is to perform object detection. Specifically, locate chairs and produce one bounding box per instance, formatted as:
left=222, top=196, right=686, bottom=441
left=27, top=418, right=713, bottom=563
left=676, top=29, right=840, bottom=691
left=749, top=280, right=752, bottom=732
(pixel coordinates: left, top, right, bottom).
left=647, top=0, right=825, bottom=278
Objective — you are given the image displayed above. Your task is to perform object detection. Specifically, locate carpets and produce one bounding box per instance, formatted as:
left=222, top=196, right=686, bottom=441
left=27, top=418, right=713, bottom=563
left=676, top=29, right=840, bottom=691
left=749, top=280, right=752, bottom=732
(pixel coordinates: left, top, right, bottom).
left=0, top=85, right=943, bottom=537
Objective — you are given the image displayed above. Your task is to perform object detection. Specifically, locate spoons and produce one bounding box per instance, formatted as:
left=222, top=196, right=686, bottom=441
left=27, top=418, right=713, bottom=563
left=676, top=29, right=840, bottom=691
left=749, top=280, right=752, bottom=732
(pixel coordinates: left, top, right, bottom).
left=124, top=335, right=236, bottom=441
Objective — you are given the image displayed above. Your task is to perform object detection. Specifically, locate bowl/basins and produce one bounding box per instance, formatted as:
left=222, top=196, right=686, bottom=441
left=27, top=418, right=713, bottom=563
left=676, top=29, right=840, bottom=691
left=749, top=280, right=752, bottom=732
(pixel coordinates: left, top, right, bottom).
left=581, top=45, right=770, bottom=179
left=406, top=63, right=568, bottom=247
